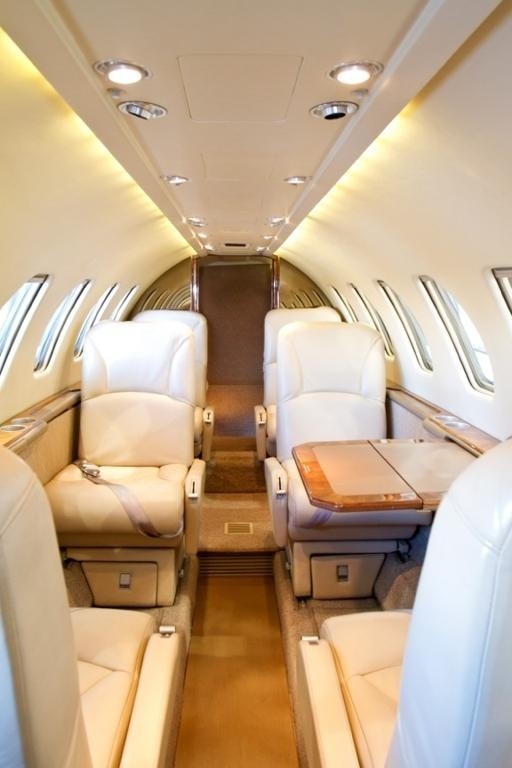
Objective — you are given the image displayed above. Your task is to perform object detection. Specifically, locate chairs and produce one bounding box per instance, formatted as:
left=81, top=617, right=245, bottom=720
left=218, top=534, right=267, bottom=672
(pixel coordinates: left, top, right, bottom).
left=0, top=446, right=185, bottom=768
left=291, top=438, right=511, bottom=767
left=253, top=307, right=341, bottom=463
left=270, top=318, right=433, bottom=536
left=133, top=309, right=214, bottom=463
left=42, top=319, right=200, bottom=551
